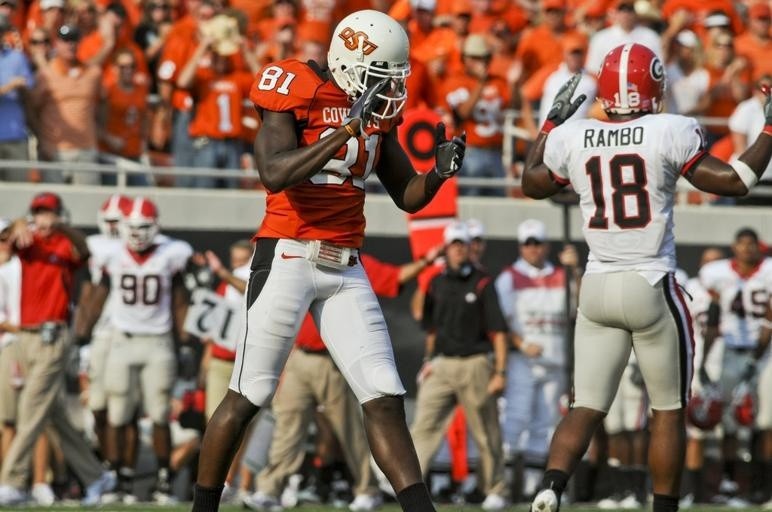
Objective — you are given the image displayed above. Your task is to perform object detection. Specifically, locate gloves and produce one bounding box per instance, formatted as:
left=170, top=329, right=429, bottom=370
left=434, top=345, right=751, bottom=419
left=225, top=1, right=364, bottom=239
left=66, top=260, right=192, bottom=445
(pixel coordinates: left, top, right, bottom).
left=341, top=77, right=392, bottom=139
left=434, top=122, right=466, bottom=180
left=541, top=74, right=586, bottom=133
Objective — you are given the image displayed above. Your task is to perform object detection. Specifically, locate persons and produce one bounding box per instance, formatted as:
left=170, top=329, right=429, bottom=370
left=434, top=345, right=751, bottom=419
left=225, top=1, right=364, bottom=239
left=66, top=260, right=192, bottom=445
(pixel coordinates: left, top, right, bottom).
left=2, top=0, right=772, bottom=511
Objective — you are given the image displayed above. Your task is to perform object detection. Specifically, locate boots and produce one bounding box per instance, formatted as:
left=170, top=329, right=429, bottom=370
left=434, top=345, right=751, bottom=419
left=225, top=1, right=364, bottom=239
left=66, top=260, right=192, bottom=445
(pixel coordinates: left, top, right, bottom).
left=104, top=466, right=179, bottom=505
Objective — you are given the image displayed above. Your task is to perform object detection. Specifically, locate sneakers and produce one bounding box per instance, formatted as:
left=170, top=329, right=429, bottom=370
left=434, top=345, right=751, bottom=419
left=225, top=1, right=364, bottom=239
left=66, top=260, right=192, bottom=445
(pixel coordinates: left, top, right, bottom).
left=242, top=492, right=281, bottom=511
left=598, top=478, right=770, bottom=509
left=482, top=493, right=503, bottom=510
left=348, top=490, right=381, bottom=511
left=0, top=485, right=31, bottom=506
left=32, top=479, right=56, bottom=506
left=529, top=477, right=561, bottom=512
left=84, top=473, right=110, bottom=505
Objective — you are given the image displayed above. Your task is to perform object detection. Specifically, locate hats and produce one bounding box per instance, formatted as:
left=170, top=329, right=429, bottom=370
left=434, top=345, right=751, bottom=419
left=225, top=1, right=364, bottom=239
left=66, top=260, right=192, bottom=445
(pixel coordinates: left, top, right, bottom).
left=32, top=194, right=62, bottom=212
left=519, top=220, right=546, bottom=243
left=444, top=218, right=489, bottom=244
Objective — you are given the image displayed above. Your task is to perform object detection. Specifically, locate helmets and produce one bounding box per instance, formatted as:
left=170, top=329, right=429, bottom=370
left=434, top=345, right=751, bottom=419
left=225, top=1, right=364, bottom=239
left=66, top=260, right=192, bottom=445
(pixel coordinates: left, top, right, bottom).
left=327, top=9, right=410, bottom=120
left=97, top=195, right=158, bottom=250
left=730, top=382, right=758, bottom=426
left=688, top=395, right=723, bottom=431
left=598, top=43, right=664, bottom=116
